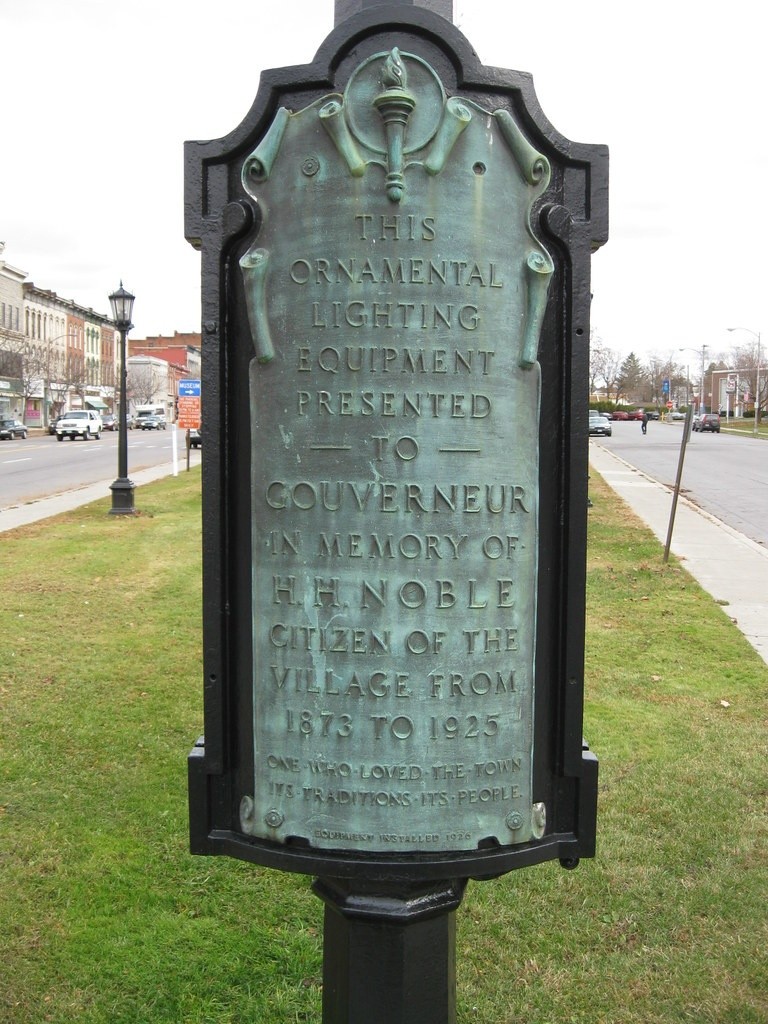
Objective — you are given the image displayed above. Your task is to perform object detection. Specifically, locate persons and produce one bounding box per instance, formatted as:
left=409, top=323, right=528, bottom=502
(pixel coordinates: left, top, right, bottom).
left=641, top=413, right=648, bottom=435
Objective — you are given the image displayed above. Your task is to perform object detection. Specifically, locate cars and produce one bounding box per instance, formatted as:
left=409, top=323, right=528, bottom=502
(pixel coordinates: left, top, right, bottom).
left=692, top=416, right=700, bottom=430
left=49, top=414, right=63, bottom=434
left=141, top=416, right=167, bottom=431
left=101, top=415, right=119, bottom=431
left=0, top=418, right=28, bottom=441
left=672, top=412, right=683, bottom=420
left=136, top=414, right=156, bottom=427
left=589, top=417, right=612, bottom=437
left=185, top=429, right=202, bottom=449
left=125, top=413, right=136, bottom=429
left=589, top=410, right=660, bottom=421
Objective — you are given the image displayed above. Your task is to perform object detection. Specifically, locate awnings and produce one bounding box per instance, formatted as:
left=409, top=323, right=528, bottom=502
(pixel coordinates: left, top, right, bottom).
left=84, top=401, right=110, bottom=411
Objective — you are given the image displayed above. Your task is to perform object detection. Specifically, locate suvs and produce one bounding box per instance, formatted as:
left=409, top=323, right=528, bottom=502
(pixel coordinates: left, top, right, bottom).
left=695, top=414, right=720, bottom=433
left=56, top=410, right=103, bottom=441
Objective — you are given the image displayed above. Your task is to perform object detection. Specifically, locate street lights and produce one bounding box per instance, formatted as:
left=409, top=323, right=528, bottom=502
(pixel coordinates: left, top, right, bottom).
left=679, top=344, right=705, bottom=413
left=43, top=334, right=76, bottom=428
left=108, top=277, right=136, bottom=517
left=726, top=326, right=760, bottom=437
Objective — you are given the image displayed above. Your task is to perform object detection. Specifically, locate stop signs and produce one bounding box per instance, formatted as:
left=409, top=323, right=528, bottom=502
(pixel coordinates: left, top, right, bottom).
left=666, top=401, right=674, bottom=408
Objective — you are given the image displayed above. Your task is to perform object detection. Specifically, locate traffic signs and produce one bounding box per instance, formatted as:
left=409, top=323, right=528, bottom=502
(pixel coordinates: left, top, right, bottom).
left=178, top=380, right=201, bottom=397
